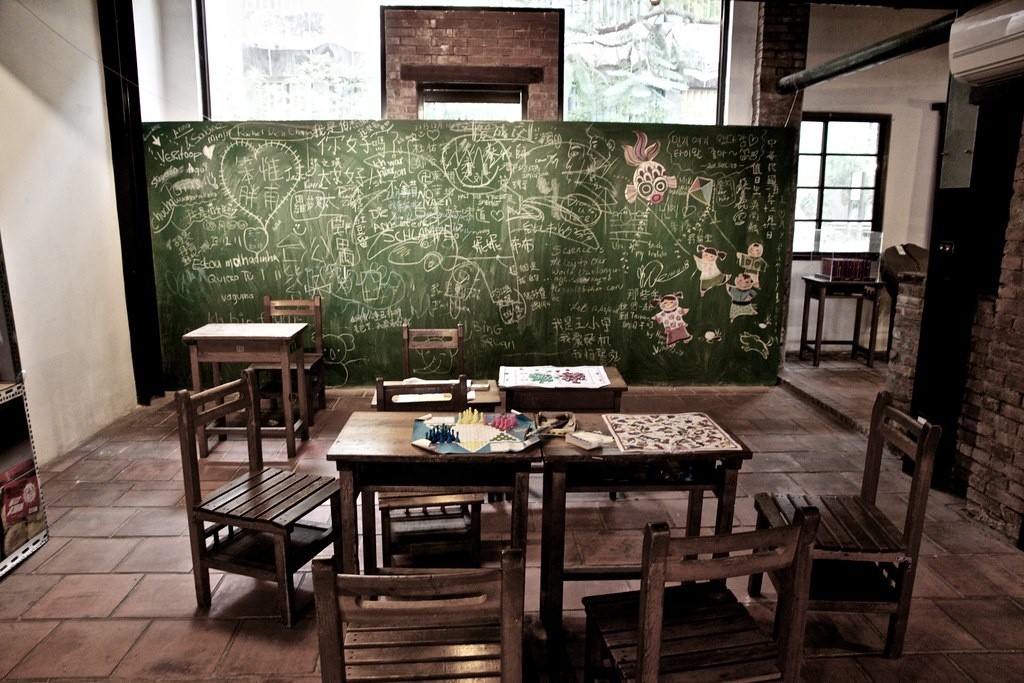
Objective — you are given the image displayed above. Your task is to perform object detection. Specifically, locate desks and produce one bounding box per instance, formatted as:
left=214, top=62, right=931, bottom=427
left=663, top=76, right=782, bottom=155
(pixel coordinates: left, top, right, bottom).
left=183, top=323, right=309, bottom=458
left=535, top=412, right=752, bottom=626
left=327, top=412, right=543, bottom=603
left=369, top=379, right=502, bottom=503
left=498, top=366, right=628, bottom=502
left=801, top=276, right=885, bottom=367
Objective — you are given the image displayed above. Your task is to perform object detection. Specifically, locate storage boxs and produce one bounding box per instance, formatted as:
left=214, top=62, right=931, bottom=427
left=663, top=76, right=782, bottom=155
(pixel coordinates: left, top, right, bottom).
left=809, top=228, right=884, bottom=282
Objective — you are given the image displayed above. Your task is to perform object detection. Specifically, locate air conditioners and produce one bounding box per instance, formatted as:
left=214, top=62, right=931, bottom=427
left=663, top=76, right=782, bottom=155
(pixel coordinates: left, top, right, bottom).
left=950, top=0, right=1024, bottom=86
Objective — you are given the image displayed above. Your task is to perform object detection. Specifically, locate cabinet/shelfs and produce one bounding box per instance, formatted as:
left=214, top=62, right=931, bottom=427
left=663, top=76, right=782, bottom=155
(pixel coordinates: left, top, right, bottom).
left=0, top=235, right=49, bottom=582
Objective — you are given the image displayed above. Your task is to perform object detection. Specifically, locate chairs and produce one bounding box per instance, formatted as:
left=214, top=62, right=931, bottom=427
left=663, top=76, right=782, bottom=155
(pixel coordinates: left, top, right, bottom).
left=175, top=366, right=342, bottom=629
left=249, top=295, right=326, bottom=427
left=376, top=375, right=485, bottom=567
left=403, top=323, right=465, bottom=378
left=312, top=550, right=526, bottom=683
left=583, top=505, right=821, bottom=683
left=748, top=392, right=942, bottom=658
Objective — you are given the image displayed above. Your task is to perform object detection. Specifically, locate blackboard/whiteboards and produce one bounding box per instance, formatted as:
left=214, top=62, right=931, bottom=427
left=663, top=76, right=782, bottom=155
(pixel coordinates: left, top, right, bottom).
left=142, top=120, right=796, bottom=388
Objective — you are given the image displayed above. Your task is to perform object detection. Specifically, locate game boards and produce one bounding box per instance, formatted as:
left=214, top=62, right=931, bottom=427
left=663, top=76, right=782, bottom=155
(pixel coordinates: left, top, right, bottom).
left=424, top=415, right=522, bottom=453
